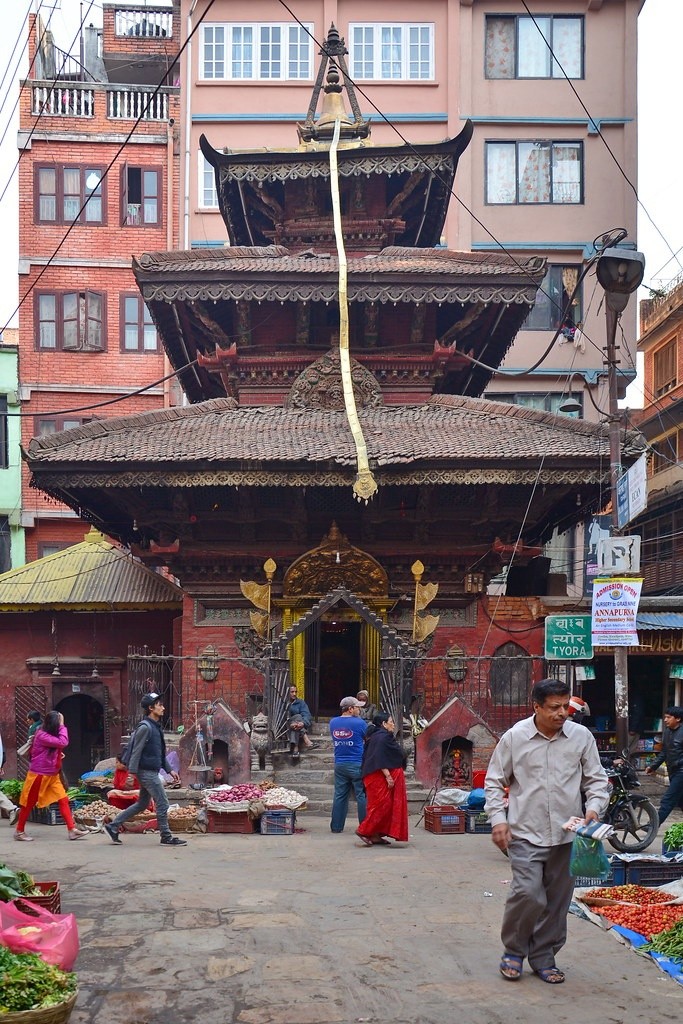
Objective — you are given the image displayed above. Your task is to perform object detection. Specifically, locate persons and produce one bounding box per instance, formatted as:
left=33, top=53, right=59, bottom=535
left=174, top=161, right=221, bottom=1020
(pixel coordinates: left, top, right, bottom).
left=0, top=709, right=90, bottom=843
left=329, top=690, right=408, bottom=845
left=483, top=678, right=610, bottom=984
left=103, top=693, right=187, bottom=847
left=639, top=707, right=683, bottom=834
left=288, top=683, right=319, bottom=758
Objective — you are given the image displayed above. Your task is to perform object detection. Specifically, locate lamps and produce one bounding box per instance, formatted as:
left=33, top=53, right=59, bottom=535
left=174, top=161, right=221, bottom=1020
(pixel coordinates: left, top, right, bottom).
left=51, top=616, right=61, bottom=675
left=90, top=614, right=100, bottom=679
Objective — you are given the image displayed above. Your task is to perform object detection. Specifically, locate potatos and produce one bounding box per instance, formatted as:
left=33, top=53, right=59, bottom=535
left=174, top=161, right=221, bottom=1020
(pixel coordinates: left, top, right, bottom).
left=75, top=799, right=200, bottom=818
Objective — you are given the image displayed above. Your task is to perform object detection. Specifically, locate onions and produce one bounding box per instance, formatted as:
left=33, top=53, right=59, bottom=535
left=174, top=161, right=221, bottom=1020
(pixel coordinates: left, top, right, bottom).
left=209, top=784, right=265, bottom=803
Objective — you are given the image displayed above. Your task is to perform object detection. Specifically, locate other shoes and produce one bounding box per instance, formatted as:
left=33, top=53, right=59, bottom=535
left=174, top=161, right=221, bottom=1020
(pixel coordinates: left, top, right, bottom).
left=14, top=831, right=33, bottom=841
left=69, top=829, right=90, bottom=839
left=292, top=751, right=300, bottom=757
left=640, top=823, right=649, bottom=831
left=308, top=742, right=319, bottom=749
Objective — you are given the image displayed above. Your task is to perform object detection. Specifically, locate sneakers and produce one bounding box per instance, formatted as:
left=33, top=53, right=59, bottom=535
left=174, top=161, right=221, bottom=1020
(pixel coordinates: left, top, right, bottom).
left=160, top=837, right=188, bottom=846
left=9, top=808, right=21, bottom=825
left=103, top=826, right=122, bottom=844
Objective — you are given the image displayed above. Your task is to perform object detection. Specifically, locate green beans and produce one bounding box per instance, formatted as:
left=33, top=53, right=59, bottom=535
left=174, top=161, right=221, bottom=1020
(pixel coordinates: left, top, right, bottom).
left=637, top=919, right=683, bottom=958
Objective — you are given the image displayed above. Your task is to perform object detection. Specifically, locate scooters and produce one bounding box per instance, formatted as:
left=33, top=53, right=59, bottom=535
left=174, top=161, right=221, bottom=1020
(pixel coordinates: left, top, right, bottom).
left=598, top=748, right=659, bottom=852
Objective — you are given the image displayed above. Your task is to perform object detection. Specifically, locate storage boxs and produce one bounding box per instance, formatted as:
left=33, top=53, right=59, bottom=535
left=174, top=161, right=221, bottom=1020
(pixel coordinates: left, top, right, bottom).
left=0, top=881, right=62, bottom=917
left=574, top=855, right=683, bottom=887
left=423, top=805, right=492, bottom=834
left=207, top=810, right=296, bottom=835
left=28, top=802, right=76, bottom=825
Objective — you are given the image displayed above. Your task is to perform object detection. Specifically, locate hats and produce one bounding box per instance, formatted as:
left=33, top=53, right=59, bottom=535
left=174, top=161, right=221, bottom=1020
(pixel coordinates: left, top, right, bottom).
left=340, top=696, right=366, bottom=707
left=140, top=693, right=165, bottom=707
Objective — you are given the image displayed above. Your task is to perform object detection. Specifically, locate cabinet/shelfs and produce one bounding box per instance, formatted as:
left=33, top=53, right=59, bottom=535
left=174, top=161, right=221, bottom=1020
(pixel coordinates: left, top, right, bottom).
left=592, top=731, right=664, bottom=752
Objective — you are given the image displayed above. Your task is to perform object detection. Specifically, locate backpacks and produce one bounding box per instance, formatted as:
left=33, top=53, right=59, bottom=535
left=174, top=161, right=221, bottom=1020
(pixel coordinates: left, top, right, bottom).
left=121, top=720, right=153, bottom=763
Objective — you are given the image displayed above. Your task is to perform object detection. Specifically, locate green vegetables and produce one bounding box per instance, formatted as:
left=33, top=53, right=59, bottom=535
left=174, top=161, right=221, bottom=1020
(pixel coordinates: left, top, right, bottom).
left=0, top=863, right=57, bottom=900
left=0, top=945, right=78, bottom=1011
left=65, top=785, right=102, bottom=802
left=0, top=778, right=25, bottom=798
left=663, top=822, right=683, bottom=853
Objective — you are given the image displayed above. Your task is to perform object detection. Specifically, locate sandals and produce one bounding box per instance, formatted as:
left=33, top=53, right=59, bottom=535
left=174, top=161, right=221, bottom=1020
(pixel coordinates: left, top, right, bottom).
left=370, top=837, right=391, bottom=844
left=355, top=830, right=373, bottom=845
left=500, top=953, right=524, bottom=980
left=535, top=965, right=565, bottom=983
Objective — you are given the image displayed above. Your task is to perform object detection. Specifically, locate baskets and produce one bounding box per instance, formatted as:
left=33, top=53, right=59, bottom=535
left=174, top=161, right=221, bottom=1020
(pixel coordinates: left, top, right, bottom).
left=74, top=816, right=101, bottom=827
left=0, top=986, right=79, bottom=1024
left=168, top=815, right=197, bottom=830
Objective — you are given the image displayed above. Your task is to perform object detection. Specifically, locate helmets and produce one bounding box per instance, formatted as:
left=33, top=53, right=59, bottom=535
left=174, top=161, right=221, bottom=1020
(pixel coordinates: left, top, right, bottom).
left=568, top=696, right=587, bottom=718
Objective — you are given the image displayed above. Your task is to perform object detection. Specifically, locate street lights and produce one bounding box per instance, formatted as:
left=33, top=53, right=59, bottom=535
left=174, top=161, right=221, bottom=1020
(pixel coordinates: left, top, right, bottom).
left=559, top=248, right=646, bottom=788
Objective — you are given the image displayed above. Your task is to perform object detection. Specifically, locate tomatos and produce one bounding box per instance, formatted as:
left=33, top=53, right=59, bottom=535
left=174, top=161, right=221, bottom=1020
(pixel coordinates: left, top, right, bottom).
left=584, top=883, right=683, bottom=939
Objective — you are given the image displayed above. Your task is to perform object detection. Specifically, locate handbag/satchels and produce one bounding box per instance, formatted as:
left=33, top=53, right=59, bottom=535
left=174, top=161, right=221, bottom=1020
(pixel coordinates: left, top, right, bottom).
left=17, top=735, right=35, bottom=761
left=569, top=834, right=611, bottom=882
left=0, top=898, right=79, bottom=972
left=61, top=771, right=69, bottom=791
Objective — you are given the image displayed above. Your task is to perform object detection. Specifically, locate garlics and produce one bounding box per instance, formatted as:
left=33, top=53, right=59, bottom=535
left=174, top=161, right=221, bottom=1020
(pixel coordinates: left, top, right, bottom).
left=261, top=787, right=304, bottom=806
